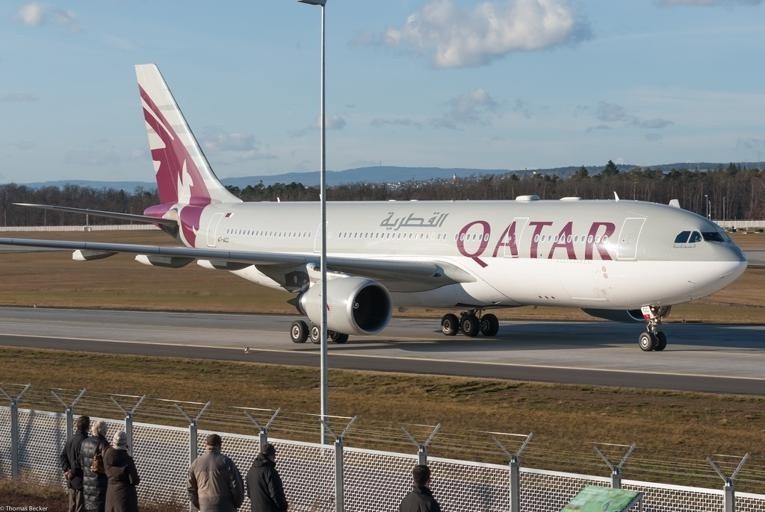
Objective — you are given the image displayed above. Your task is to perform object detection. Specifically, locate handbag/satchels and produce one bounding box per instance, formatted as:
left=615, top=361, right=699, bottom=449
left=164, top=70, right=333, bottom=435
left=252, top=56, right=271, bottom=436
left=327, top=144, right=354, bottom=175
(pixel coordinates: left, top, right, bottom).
left=91, top=456, right=104, bottom=473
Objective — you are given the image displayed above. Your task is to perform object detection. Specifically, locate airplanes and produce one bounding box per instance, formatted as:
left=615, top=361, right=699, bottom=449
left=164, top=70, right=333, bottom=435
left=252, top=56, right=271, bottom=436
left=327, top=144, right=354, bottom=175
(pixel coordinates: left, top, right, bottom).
left=10, top=62, right=748, bottom=363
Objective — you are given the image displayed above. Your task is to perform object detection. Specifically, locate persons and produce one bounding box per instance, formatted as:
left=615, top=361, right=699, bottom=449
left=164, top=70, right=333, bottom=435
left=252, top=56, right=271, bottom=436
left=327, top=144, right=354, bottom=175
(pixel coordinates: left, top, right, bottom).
left=397, top=464, right=443, bottom=511
left=184, top=432, right=245, bottom=512
left=246, top=442, right=291, bottom=511
left=58, top=413, right=91, bottom=511
left=103, top=429, right=141, bottom=511
left=77, top=420, right=109, bottom=512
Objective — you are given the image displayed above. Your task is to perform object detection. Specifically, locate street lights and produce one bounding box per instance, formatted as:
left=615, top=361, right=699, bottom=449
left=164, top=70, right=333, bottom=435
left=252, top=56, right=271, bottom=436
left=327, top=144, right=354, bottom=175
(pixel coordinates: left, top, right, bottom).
left=299, top=0, right=334, bottom=457
left=701, top=194, right=728, bottom=223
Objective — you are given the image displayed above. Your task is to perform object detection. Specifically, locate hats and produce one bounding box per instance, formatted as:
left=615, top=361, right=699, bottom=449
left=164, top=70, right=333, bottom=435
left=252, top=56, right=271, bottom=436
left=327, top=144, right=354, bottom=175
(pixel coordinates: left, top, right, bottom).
left=77, top=416, right=90, bottom=430
left=92, top=420, right=107, bottom=437
left=113, top=432, right=127, bottom=446
left=207, top=434, right=222, bottom=446
left=262, top=445, right=275, bottom=455
